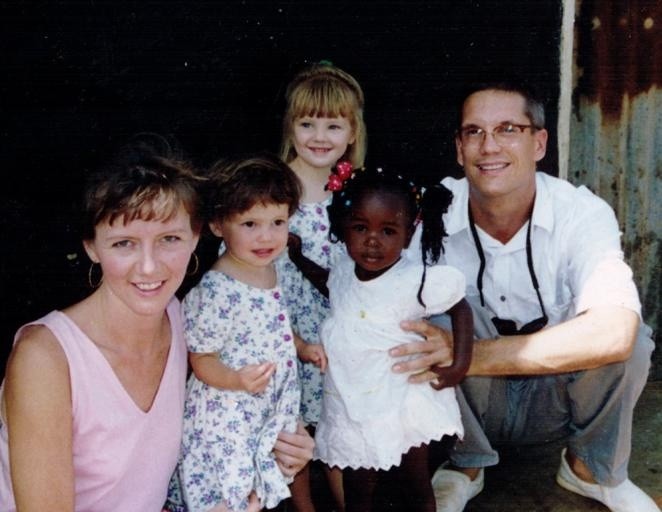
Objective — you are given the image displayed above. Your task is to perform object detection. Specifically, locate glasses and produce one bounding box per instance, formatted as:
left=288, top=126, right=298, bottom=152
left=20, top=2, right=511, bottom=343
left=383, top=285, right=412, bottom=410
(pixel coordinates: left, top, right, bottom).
left=463, top=121, right=537, bottom=145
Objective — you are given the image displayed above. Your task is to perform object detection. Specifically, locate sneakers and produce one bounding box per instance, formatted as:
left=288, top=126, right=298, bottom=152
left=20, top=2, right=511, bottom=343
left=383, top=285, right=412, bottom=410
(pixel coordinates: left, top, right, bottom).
left=555, top=446, right=661, bottom=512
left=432, top=461, right=485, bottom=511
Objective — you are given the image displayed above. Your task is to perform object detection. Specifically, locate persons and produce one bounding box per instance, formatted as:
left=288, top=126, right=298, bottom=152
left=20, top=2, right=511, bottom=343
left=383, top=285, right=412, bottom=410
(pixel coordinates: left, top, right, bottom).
left=163, top=152, right=329, bottom=512
left=214, top=60, right=374, bottom=512
left=0, top=148, right=206, bottom=512
left=390, top=78, right=662, bottom=512
left=304, top=159, right=473, bottom=512
